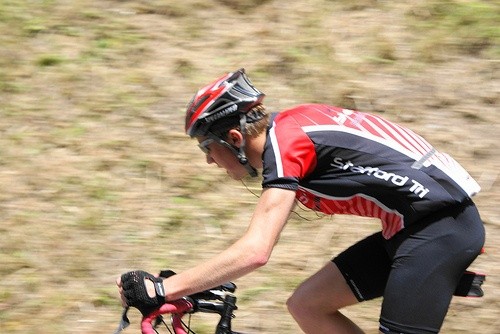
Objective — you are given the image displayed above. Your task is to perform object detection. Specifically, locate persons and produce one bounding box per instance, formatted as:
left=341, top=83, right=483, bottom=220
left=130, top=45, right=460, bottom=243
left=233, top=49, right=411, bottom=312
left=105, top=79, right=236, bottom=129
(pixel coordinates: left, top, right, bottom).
left=116, top=68, right=486, bottom=334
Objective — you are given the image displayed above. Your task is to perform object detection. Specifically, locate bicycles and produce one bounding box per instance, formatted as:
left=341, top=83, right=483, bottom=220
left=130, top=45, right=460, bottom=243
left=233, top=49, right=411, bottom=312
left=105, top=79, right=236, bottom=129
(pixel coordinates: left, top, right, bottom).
left=115, top=247, right=499, bottom=334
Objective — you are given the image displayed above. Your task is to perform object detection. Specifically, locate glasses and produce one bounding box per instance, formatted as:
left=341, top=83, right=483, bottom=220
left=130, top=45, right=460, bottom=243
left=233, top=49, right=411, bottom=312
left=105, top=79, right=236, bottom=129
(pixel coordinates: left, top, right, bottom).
left=198, top=135, right=225, bottom=153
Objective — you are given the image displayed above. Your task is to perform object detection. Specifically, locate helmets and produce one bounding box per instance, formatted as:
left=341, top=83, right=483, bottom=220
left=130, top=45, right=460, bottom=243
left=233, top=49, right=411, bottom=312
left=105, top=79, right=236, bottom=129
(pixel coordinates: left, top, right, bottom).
left=185, top=69, right=264, bottom=138
